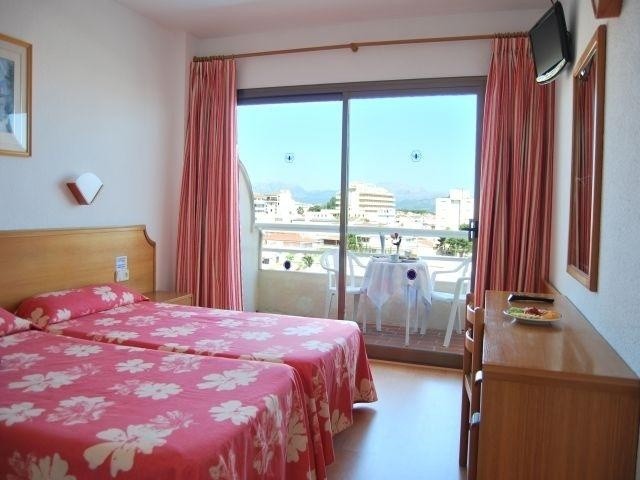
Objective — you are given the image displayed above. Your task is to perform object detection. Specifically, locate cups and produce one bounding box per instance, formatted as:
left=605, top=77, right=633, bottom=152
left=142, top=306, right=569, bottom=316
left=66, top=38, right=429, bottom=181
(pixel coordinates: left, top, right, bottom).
left=388, top=253, right=401, bottom=262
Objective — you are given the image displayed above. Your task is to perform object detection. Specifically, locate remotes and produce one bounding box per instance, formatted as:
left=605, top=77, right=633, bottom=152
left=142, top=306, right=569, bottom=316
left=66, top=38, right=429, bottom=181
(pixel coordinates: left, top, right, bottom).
left=508, top=293, right=554, bottom=304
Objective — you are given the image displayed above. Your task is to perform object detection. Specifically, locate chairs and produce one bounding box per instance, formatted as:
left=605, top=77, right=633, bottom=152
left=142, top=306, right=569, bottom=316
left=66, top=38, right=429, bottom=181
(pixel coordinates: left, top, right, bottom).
left=421, top=257, right=472, bottom=344
left=459, top=292, right=487, bottom=480
left=320, top=249, right=384, bottom=333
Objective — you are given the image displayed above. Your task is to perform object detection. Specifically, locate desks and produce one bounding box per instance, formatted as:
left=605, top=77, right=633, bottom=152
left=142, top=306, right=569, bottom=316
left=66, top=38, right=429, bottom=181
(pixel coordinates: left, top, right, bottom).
left=362, top=254, right=434, bottom=337
left=478, top=288, right=639, bottom=478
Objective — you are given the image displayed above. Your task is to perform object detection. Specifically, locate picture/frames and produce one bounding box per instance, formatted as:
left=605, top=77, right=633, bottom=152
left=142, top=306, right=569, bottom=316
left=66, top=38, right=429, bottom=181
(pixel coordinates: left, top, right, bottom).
left=0, top=32, right=33, bottom=158
left=568, top=26, right=607, bottom=290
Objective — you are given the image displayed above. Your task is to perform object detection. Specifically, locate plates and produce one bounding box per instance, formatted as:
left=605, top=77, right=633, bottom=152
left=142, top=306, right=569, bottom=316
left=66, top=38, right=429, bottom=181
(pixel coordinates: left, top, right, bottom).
left=502, top=308, right=563, bottom=325
left=502, top=321, right=564, bottom=334
left=370, top=256, right=388, bottom=262
left=401, top=258, right=420, bottom=263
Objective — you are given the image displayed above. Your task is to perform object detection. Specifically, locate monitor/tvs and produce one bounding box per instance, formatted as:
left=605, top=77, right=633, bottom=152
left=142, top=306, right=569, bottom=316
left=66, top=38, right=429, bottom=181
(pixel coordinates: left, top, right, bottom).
left=527, top=1, right=573, bottom=85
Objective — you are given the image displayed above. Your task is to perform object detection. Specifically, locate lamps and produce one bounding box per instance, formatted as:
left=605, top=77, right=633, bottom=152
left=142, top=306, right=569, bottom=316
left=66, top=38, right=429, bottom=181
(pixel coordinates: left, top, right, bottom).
left=68, top=172, right=105, bottom=206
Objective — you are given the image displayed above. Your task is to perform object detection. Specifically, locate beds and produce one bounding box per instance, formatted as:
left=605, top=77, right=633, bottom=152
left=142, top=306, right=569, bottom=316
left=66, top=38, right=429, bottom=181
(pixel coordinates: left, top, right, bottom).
left=0, top=330, right=294, bottom=478
left=6, top=298, right=377, bottom=438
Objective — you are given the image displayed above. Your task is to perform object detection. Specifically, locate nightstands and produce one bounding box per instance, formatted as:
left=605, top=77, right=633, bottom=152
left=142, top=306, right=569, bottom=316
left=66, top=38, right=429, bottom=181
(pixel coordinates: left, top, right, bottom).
left=143, top=290, right=193, bottom=306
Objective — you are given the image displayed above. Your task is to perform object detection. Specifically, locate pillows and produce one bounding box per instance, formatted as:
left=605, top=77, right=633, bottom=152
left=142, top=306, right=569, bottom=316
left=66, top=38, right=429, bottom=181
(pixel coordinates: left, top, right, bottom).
left=15, top=287, right=149, bottom=329
left=0, top=307, right=40, bottom=338
